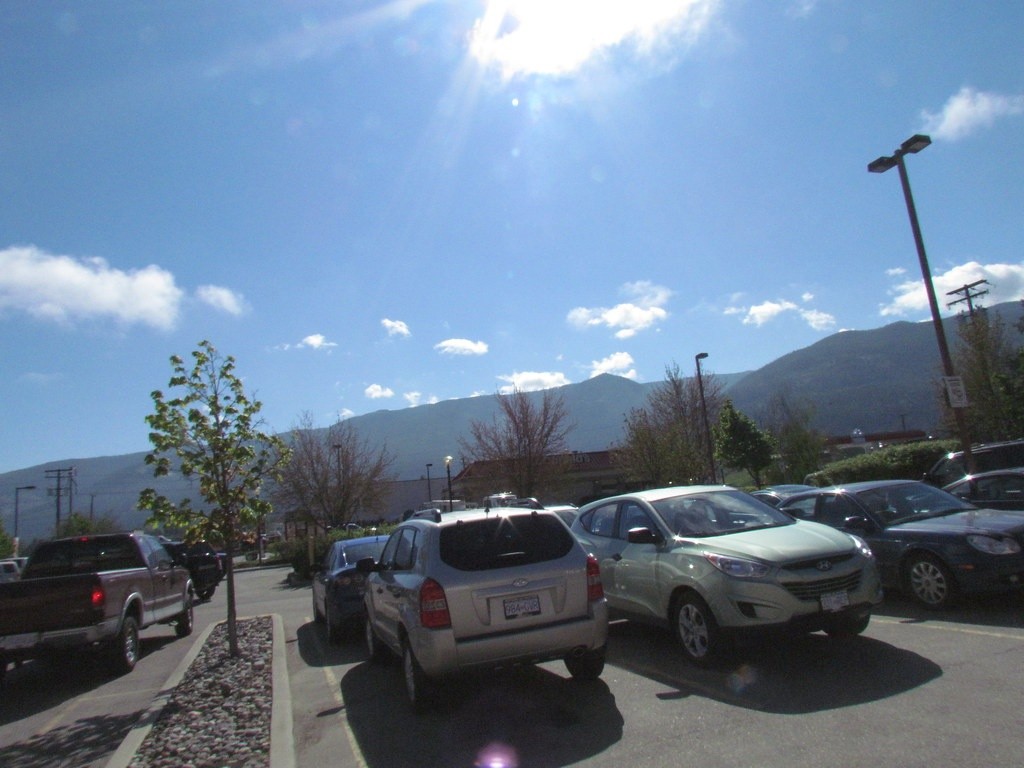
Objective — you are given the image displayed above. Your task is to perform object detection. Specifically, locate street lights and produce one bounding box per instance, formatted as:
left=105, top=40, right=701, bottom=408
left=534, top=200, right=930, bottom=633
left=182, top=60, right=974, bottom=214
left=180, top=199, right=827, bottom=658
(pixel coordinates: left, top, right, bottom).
left=15, top=485, right=37, bottom=537
left=695, top=352, right=717, bottom=484
left=426, top=462, right=432, bottom=502
left=868, top=135, right=975, bottom=475
left=443, top=456, right=453, bottom=512
left=254, top=486, right=263, bottom=566
left=332, top=443, right=341, bottom=494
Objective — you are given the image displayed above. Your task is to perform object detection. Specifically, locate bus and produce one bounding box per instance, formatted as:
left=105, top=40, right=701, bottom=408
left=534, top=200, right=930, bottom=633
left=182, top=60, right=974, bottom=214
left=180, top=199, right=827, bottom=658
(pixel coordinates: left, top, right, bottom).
left=483, top=492, right=517, bottom=508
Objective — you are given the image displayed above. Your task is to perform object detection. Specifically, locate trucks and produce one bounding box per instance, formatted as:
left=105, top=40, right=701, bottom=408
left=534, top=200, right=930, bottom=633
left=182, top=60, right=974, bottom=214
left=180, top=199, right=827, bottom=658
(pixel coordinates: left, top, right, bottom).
left=419, top=500, right=465, bottom=514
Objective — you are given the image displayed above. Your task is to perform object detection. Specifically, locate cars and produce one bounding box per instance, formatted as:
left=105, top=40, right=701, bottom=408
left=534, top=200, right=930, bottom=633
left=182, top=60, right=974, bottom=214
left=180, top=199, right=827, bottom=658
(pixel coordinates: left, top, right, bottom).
left=726, top=433, right=1024, bottom=615
left=326, top=525, right=375, bottom=535
left=0, top=531, right=281, bottom=599
left=308, top=534, right=405, bottom=649
left=501, top=485, right=876, bottom=670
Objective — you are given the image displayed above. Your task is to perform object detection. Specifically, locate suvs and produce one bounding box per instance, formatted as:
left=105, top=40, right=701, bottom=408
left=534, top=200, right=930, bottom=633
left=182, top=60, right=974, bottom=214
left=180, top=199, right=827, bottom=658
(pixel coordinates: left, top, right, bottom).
left=356, top=496, right=607, bottom=714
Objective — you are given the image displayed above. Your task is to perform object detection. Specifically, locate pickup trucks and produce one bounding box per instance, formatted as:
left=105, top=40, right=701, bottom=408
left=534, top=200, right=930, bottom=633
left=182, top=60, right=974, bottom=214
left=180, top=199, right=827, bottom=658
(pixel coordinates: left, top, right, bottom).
left=0, top=533, right=194, bottom=676
